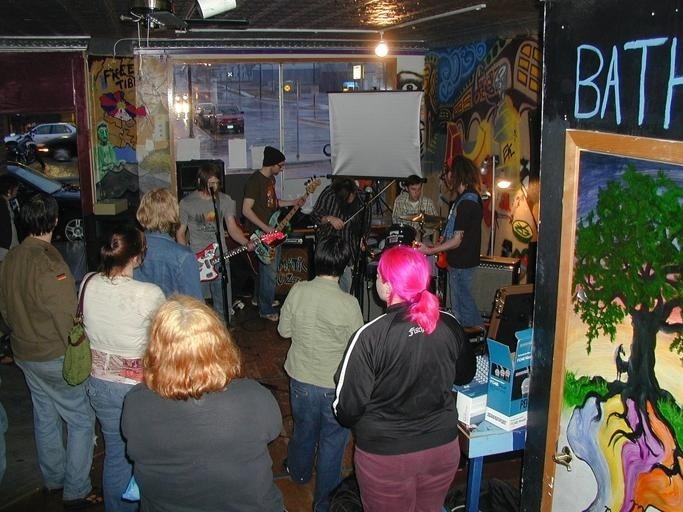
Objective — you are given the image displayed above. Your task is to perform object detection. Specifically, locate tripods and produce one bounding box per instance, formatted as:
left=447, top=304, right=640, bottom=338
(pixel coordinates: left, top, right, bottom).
left=370, top=180, right=394, bottom=217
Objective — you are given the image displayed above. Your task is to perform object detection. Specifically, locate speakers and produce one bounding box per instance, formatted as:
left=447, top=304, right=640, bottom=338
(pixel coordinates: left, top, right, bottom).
left=363, top=255, right=439, bottom=323
left=177, top=159, right=224, bottom=202
left=222, top=232, right=259, bottom=297
left=225, top=169, right=260, bottom=229
left=446, top=255, right=520, bottom=325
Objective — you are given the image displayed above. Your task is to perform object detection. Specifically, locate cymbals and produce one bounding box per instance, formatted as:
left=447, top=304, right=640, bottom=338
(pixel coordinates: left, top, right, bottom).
left=406, top=213, right=447, bottom=224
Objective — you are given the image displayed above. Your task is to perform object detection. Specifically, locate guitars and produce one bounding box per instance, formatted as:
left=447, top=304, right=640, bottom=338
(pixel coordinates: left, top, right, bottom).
left=411, top=236, right=448, bottom=269
left=249, top=175, right=321, bottom=265
left=195, top=230, right=286, bottom=282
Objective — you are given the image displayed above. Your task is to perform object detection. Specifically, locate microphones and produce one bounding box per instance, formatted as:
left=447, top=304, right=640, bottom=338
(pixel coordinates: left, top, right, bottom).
left=364, top=187, right=372, bottom=205
left=209, top=185, right=216, bottom=203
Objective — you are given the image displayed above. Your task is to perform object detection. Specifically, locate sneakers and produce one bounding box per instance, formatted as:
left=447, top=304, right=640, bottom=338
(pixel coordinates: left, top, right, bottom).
left=43, top=487, right=64, bottom=496
left=61, top=493, right=104, bottom=507
left=250, top=295, right=281, bottom=309
left=0, top=353, right=15, bottom=365
left=259, top=313, right=279, bottom=322
left=463, top=326, right=482, bottom=339
left=283, top=456, right=290, bottom=475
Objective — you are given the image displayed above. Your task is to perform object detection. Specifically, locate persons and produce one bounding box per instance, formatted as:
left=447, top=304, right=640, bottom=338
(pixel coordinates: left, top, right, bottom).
left=96, top=121, right=126, bottom=173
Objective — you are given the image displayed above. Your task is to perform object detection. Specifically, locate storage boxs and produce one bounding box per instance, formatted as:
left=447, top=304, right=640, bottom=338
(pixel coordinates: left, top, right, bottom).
left=478, top=328, right=535, bottom=432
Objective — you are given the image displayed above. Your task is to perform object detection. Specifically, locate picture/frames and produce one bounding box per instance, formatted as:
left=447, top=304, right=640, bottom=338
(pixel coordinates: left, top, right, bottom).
left=538, top=131, right=683, bottom=511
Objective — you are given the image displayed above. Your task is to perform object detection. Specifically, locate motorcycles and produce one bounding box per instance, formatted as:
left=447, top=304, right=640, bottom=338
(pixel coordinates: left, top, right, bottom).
left=5, top=128, right=47, bottom=170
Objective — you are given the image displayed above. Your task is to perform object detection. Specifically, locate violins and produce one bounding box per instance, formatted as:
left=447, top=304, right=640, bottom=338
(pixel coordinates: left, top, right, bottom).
left=343, top=198, right=369, bottom=235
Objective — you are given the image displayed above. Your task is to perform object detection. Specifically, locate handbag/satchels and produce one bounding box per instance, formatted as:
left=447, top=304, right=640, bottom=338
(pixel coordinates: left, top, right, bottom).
left=59, top=272, right=108, bottom=386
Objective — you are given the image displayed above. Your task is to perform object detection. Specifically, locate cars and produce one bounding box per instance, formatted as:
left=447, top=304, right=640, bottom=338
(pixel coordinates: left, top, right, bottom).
left=174, top=89, right=247, bottom=135
left=4, top=120, right=77, bottom=156
left=1, top=161, right=85, bottom=243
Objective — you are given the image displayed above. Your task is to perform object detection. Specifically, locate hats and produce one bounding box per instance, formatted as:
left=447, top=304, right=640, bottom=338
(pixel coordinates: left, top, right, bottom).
left=261, top=145, right=286, bottom=166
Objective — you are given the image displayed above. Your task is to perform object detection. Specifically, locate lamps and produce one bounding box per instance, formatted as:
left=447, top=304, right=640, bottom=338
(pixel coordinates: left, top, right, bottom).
left=496, top=177, right=539, bottom=239
left=374, top=28, right=389, bottom=60
left=478, top=152, right=496, bottom=259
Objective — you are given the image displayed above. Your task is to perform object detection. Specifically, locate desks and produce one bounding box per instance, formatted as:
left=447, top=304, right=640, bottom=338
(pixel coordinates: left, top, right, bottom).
left=456, top=418, right=528, bottom=511
